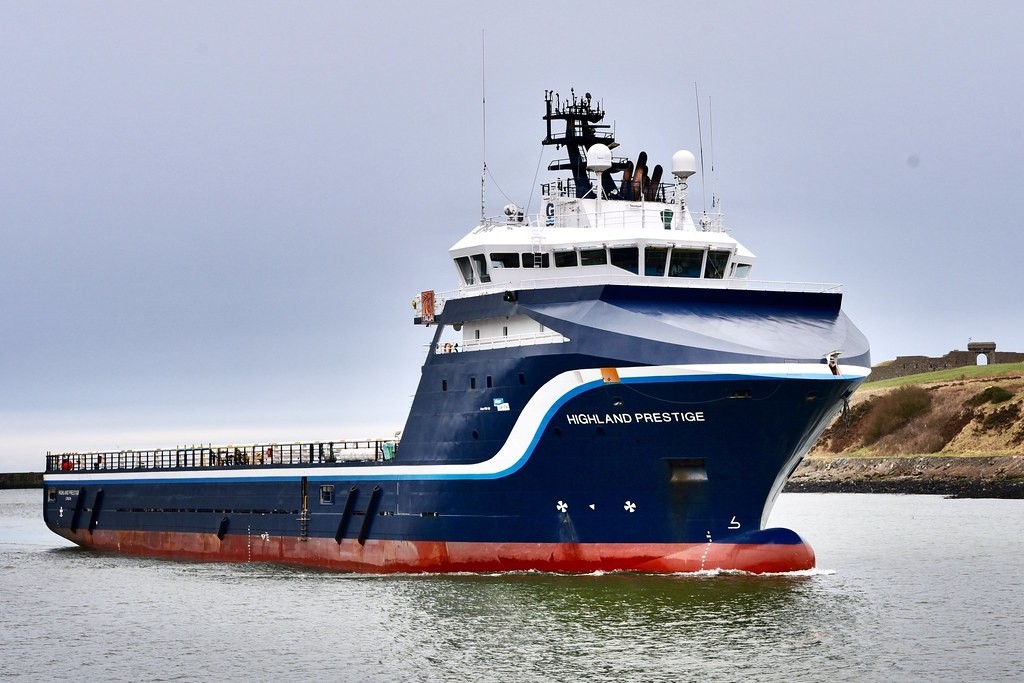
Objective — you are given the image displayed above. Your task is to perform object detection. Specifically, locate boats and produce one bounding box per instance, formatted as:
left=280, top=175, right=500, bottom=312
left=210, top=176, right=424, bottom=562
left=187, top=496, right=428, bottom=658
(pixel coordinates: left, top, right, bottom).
left=38, top=87, right=873, bottom=578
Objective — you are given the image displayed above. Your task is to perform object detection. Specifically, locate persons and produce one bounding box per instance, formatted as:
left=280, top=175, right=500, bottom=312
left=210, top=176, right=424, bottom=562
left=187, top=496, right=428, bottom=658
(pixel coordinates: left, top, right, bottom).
left=53, top=448, right=273, bottom=471
